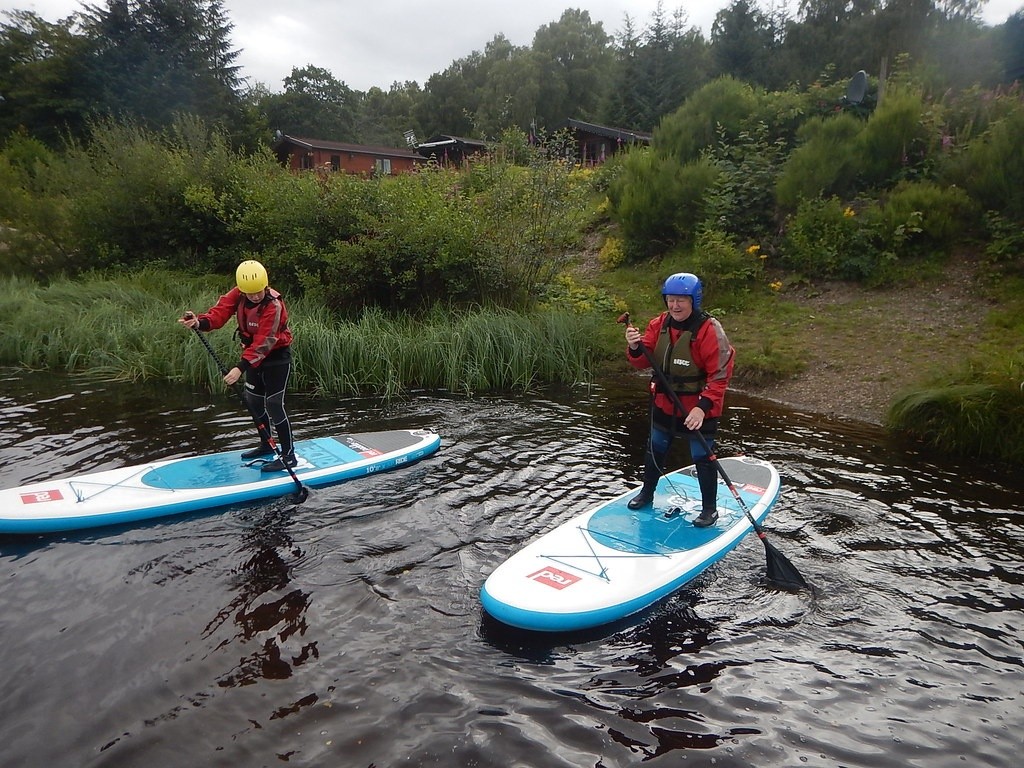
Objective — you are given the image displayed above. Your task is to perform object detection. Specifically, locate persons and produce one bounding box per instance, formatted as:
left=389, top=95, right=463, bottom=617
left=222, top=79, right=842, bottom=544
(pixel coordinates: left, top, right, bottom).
left=626, top=272, right=736, bottom=528
left=177, top=260, right=298, bottom=473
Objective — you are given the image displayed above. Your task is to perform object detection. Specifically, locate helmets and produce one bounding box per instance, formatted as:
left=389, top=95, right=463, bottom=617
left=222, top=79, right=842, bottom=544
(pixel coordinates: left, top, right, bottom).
left=662, top=273, right=702, bottom=310
left=236, top=260, right=269, bottom=293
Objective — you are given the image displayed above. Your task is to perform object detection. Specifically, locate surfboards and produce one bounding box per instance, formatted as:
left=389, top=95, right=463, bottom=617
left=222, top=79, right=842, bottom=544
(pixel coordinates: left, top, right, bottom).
left=0, top=426, right=441, bottom=533
left=477, top=457, right=782, bottom=632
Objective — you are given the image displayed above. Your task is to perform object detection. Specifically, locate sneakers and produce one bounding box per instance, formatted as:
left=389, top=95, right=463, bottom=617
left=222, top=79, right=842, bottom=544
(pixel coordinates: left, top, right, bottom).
left=694, top=510, right=718, bottom=526
left=261, top=454, right=297, bottom=472
left=241, top=445, right=278, bottom=457
left=628, top=493, right=653, bottom=510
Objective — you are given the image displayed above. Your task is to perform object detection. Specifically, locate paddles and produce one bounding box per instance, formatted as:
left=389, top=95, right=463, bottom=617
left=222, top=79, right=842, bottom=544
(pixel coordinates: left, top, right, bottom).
left=184, top=311, right=309, bottom=504
left=615, top=308, right=810, bottom=588
